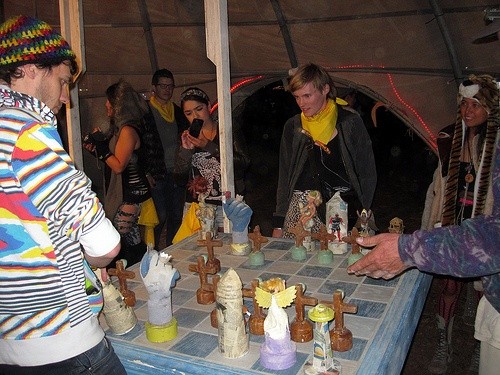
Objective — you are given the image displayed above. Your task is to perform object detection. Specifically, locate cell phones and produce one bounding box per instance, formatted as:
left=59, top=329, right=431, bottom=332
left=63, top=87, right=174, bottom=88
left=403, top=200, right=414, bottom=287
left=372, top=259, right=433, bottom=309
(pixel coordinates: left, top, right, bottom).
left=189, top=118, right=204, bottom=138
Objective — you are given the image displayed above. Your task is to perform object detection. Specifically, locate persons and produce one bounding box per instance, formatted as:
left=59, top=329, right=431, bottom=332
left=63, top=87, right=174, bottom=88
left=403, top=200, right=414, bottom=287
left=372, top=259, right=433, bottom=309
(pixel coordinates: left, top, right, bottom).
left=420, top=74, right=500, bottom=375
left=253, top=279, right=297, bottom=370
left=347, top=125, right=500, bottom=375
left=341, top=88, right=384, bottom=146
left=175, top=87, right=248, bottom=231
left=356, top=209, right=371, bottom=227
left=299, top=190, right=323, bottom=252
left=328, top=213, right=344, bottom=243
left=0, top=14, right=126, bottom=375
left=81, top=82, right=160, bottom=269
left=143, top=69, right=191, bottom=252
left=271, top=62, right=378, bottom=240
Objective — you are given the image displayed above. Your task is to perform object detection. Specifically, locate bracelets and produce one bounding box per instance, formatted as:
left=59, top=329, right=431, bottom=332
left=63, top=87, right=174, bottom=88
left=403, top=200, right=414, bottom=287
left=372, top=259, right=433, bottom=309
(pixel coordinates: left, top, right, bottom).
left=272, top=227, right=282, bottom=230
left=102, top=152, right=111, bottom=160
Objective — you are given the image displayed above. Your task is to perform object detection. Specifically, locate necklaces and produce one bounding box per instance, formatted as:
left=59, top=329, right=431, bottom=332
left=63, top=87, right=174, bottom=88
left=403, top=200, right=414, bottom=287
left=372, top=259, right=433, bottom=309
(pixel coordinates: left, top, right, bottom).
left=464, top=131, right=483, bottom=184
left=210, top=121, right=214, bottom=140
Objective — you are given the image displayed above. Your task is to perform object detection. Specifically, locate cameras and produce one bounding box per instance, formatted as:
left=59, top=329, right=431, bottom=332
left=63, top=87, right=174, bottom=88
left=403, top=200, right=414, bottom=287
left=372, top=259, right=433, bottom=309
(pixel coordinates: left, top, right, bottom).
left=82, top=132, right=108, bottom=152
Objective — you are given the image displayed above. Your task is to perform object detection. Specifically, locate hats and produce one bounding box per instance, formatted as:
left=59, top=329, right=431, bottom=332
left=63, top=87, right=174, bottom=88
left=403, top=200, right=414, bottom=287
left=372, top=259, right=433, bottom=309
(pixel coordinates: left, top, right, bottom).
left=0, top=12, right=77, bottom=63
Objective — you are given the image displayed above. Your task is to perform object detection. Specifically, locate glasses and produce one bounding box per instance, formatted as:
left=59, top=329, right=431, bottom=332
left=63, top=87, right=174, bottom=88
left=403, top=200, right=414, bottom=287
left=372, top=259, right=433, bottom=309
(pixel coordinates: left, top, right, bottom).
left=156, top=83, right=174, bottom=88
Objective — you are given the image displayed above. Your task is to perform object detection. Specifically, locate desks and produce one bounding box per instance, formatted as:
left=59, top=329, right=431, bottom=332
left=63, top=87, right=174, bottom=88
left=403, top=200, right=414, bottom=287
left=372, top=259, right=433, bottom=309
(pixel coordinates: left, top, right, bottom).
left=99, top=232, right=432, bottom=375
